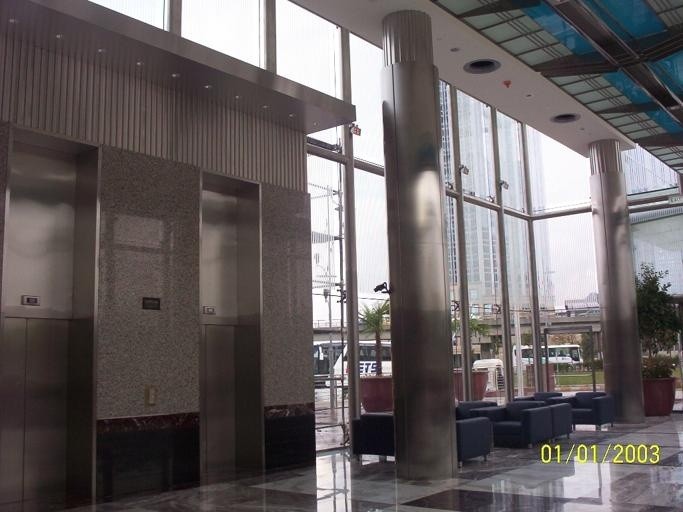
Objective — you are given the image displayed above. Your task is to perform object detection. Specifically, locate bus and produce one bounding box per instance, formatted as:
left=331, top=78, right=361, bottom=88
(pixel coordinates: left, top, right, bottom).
left=512, top=344, right=583, bottom=372
left=313, top=341, right=393, bottom=400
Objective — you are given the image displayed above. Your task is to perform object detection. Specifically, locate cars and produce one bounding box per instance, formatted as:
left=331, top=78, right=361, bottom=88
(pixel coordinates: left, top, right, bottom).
left=578, top=309, right=601, bottom=317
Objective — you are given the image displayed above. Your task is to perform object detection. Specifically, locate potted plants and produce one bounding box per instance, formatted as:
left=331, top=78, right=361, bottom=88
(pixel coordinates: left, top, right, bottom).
left=635, top=262, right=683, bottom=417
left=451, top=313, right=490, bottom=401
left=358, top=297, right=394, bottom=413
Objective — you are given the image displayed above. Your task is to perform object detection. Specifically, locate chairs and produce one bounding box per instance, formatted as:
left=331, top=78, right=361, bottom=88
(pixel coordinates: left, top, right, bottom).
left=353, top=391, right=614, bottom=468
